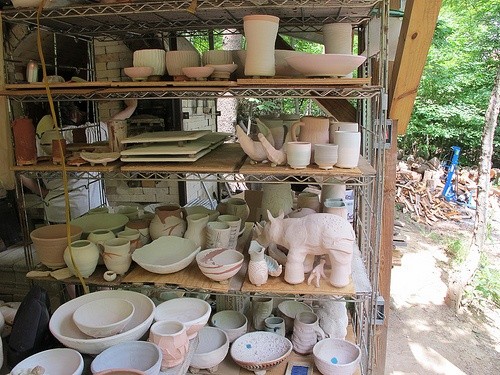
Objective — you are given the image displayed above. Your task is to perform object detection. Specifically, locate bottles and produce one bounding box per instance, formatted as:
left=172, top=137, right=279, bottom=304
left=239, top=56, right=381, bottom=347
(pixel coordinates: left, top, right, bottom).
left=148, top=205, right=188, bottom=239
left=12, top=118, right=35, bottom=160
left=87, top=229, right=116, bottom=265
left=216, top=198, right=250, bottom=231
left=247, top=240, right=269, bottom=286
left=27, top=58, right=38, bottom=83
left=64, top=239, right=100, bottom=278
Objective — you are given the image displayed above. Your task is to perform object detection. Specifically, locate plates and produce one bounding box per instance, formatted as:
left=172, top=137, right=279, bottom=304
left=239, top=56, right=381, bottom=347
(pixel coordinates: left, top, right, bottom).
left=277, top=300, right=313, bottom=319
left=80, top=129, right=233, bottom=164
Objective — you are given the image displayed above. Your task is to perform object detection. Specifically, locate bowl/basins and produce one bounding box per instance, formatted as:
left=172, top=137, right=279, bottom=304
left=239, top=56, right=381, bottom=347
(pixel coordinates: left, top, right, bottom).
left=30, top=224, right=83, bottom=268
left=195, top=247, right=246, bottom=282
left=125, top=48, right=366, bottom=82
left=70, top=213, right=129, bottom=239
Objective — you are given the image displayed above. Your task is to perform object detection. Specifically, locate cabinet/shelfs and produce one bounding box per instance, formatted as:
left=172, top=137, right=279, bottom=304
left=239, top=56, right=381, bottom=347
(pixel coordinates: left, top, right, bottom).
left=0, top=0, right=391, bottom=375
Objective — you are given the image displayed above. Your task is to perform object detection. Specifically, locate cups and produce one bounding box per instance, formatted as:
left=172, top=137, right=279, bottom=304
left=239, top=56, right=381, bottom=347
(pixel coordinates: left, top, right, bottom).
left=8, top=291, right=362, bottom=375
left=243, top=14, right=279, bottom=77
left=323, top=23, right=352, bottom=79
left=251, top=295, right=275, bottom=330
left=259, top=112, right=362, bottom=171
left=289, top=310, right=325, bottom=355
left=51, top=139, right=66, bottom=157
left=64, top=184, right=348, bottom=274
left=264, top=317, right=285, bottom=337
left=147, top=321, right=188, bottom=367
